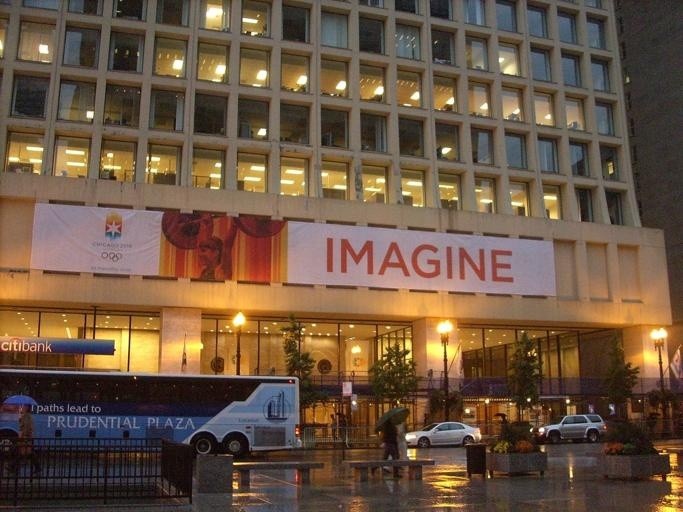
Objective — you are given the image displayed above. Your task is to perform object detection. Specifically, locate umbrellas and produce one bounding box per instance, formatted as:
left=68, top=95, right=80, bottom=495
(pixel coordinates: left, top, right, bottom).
left=495, top=413, right=507, bottom=422
left=2, top=391, right=39, bottom=421
left=375, top=406, right=410, bottom=432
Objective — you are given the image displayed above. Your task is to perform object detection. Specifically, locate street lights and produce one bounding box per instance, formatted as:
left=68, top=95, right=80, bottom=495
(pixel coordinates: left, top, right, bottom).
left=231, top=310, right=247, bottom=375
left=436, top=319, right=453, bottom=422
left=650, top=327, right=675, bottom=441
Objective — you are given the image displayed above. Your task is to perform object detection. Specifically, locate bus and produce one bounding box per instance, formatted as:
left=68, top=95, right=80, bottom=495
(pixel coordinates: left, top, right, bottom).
left=0, top=369, right=303, bottom=461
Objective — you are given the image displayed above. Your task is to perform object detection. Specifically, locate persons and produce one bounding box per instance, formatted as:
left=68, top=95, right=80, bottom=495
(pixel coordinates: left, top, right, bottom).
left=3, top=404, right=41, bottom=473
left=382, top=420, right=402, bottom=478
left=330, top=413, right=349, bottom=441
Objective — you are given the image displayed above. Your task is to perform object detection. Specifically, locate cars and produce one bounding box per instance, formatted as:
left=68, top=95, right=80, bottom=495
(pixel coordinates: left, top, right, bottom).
left=403, top=422, right=482, bottom=449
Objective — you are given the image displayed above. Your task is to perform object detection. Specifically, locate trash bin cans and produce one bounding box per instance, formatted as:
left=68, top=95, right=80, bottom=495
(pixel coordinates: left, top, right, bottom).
left=303, top=424, right=316, bottom=449
left=464, top=443, right=487, bottom=478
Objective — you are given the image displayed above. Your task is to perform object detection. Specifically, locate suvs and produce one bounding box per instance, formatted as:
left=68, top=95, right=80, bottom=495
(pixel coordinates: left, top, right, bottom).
left=529, top=413, right=607, bottom=445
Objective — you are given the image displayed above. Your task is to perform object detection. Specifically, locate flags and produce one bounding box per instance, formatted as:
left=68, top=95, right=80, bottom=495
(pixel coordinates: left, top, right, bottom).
left=669, top=346, right=683, bottom=383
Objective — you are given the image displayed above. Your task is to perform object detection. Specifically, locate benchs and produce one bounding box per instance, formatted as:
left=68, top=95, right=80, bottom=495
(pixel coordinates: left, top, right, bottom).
left=339, top=456, right=436, bottom=483
left=227, top=459, right=327, bottom=494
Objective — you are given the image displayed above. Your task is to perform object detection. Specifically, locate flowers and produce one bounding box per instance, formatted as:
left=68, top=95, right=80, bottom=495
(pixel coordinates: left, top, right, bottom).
left=601, top=433, right=659, bottom=456
left=490, top=432, right=541, bottom=454
